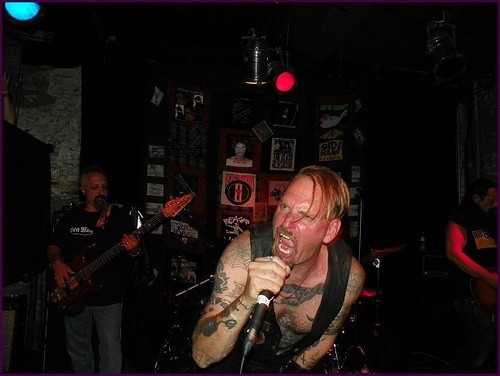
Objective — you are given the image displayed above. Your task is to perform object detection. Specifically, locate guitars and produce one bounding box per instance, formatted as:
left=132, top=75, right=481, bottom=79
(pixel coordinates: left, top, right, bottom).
left=470, top=270, right=500, bottom=313
left=46, top=189, right=198, bottom=316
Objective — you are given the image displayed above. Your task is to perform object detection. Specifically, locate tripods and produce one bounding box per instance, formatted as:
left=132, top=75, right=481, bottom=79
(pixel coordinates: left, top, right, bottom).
left=338, top=297, right=374, bottom=375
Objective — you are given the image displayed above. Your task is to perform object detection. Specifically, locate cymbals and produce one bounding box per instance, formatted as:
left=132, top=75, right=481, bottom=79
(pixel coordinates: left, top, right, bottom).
left=364, top=240, right=408, bottom=264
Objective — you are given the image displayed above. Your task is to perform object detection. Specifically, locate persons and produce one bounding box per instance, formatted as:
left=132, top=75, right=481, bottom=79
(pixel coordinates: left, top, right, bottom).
left=47, top=165, right=147, bottom=376
left=447, top=176, right=500, bottom=376
left=192, top=165, right=367, bottom=376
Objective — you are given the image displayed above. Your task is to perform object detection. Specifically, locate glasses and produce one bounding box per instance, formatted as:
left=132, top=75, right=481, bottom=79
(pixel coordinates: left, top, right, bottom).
left=81, top=183, right=109, bottom=189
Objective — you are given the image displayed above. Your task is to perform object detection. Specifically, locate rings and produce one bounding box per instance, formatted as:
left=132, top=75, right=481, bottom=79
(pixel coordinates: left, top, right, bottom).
left=270, top=256, right=274, bottom=260
left=126, top=241, right=128, bottom=245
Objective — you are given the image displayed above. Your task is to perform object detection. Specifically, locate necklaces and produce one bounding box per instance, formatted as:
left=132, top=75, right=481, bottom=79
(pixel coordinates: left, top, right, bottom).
left=278, top=257, right=323, bottom=299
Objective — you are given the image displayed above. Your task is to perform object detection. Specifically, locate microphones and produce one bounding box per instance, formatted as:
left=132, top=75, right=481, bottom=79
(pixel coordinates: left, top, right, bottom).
left=243, top=290, right=274, bottom=355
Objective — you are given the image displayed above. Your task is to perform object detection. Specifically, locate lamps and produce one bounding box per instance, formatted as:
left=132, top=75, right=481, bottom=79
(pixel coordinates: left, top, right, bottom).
left=423, top=7, right=470, bottom=84
left=236, top=26, right=268, bottom=89
left=3, top=1, right=47, bottom=25
left=269, top=45, right=300, bottom=97
